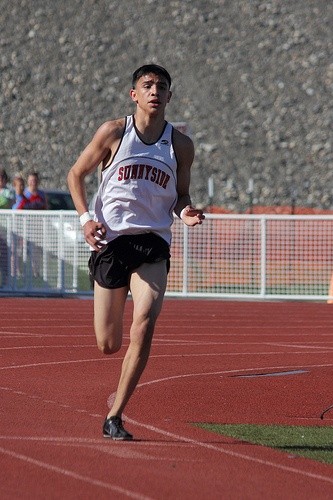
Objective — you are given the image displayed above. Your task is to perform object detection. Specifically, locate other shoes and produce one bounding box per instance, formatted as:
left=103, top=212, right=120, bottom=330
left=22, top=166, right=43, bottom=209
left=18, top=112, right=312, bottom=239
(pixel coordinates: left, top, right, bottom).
left=102, top=415, right=132, bottom=441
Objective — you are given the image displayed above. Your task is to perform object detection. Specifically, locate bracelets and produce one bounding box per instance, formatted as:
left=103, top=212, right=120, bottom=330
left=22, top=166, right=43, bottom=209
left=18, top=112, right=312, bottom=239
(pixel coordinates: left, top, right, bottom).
left=80, top=212, right=92, bottom=226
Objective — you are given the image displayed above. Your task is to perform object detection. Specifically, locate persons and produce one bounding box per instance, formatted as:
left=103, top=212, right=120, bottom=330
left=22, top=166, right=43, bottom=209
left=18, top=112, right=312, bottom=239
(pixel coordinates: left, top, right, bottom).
left=11, top=177, right=31, bottom=209
left=0, top=169, right=16, bottom=209
left=23, top=173, right=46, bottom=209
left=67, top=64, right=206, bottom=442
